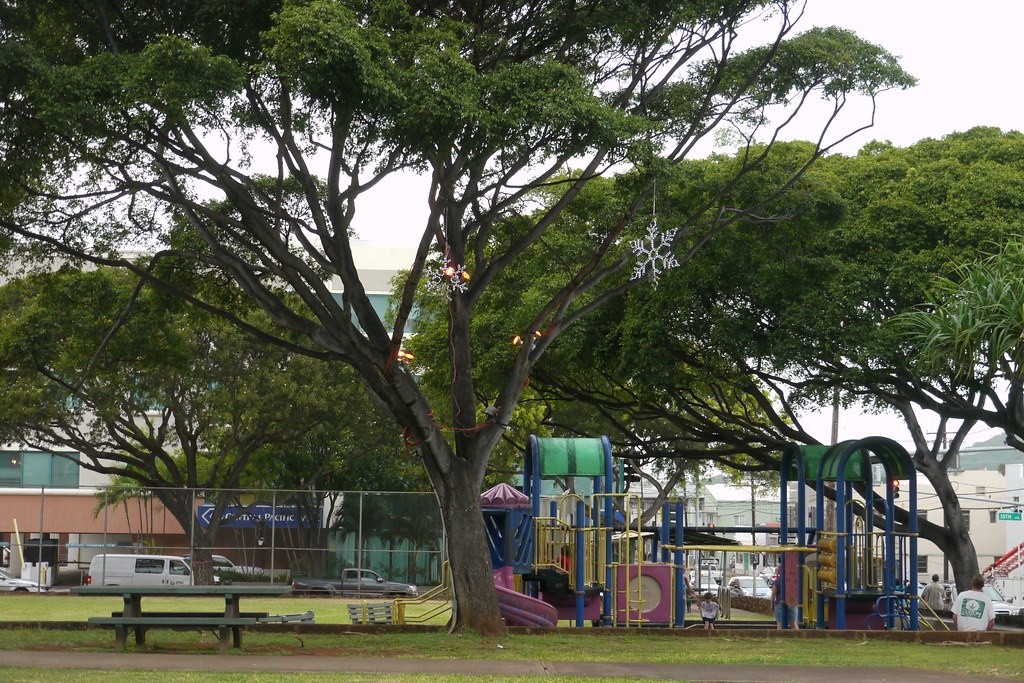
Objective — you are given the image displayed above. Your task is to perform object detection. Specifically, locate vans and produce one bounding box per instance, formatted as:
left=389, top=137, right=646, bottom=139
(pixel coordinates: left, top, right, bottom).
left=86, top=553, right=221, bottom=587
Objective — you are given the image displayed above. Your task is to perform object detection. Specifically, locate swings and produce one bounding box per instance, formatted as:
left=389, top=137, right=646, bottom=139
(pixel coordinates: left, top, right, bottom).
left=699, top=550, right=723, bottom=621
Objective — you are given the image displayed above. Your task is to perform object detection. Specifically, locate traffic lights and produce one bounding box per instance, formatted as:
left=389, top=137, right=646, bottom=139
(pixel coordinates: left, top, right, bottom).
left=893, top=480, right=900, bottom=499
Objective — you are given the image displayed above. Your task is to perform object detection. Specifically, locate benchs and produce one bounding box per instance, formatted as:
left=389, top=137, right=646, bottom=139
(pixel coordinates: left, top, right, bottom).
left=111, top=612, right=268, bottom=618
left=88, top=616, right=257, bottom=625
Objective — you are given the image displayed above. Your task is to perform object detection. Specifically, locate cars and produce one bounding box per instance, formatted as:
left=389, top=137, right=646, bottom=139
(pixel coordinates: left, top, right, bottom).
left=758, top=566, right=781, bottom=588
left=211, top=555, right=263, bottom=576
left=939, top=580, right=1024, bottom=617
left=727, top=576, right=772, bottom=600
left=689, top=577, right=720, bottom=598
left=0, top=568, right=52, bottom=592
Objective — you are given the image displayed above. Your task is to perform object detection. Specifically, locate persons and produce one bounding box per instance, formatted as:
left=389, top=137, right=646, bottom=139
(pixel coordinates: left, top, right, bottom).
left=894, top=579, right=910, bottom=606
left=697, top=593, right=722, bottom=630
left=921, top=574, right=947, bottom=609
left=547, top=546, right=570, bottom=571
left=771, top=566, right=798, bottom=629
left=683, top=566, right=697, bottom=598
left=951, top=574, right=996, bottom=631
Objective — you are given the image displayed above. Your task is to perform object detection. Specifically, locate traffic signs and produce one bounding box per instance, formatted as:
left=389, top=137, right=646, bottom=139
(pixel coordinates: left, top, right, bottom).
left=701, top=558, right=720, bottom=565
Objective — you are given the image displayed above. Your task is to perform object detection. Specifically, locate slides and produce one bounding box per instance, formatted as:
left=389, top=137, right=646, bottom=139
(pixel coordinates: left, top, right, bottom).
left=490, top=568, right=558, bottom=627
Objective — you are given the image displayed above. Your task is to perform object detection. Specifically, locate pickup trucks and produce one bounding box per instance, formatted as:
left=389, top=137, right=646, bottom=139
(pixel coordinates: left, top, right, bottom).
left=688, top=563, right=723, bottom=585
left=290, top=568, right=419, bottom=598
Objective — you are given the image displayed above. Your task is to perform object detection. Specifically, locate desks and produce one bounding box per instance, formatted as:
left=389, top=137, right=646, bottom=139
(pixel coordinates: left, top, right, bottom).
left=70, top=585, right=292, bottom=653
